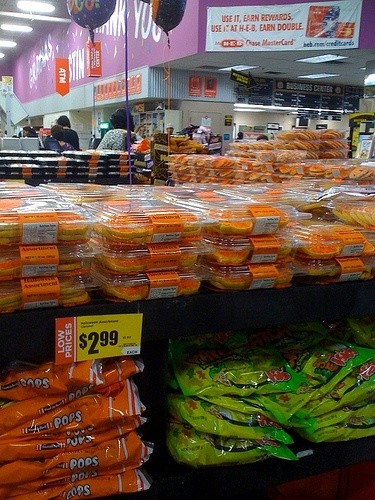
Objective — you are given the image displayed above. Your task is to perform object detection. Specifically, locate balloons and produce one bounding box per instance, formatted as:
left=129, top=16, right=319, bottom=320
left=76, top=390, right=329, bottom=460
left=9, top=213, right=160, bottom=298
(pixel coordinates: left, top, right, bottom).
left=152, top=0, right=187, bottom=36
left=66, top=0, right=116, bottom=30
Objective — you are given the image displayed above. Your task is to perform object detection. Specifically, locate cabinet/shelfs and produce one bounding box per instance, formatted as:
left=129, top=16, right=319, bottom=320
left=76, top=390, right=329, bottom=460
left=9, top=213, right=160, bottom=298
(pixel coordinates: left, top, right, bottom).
left=0, top=279, right=375, bottom=500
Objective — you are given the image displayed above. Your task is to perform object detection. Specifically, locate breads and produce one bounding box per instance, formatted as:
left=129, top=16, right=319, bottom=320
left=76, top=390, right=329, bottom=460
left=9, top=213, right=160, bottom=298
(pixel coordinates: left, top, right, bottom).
left=0, top=129, right=375, bottom=311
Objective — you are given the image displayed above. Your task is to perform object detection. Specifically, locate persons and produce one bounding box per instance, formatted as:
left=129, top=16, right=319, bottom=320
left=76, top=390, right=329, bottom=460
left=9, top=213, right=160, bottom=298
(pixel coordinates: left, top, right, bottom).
left=237, top=132, right=243, bottom=139
left=96, top=109, right=143, bottom=150
left=167, top=123, right=173, bottom=134
left=52, top=115, right=79, bottom=150
left=51, top=125, right=77, bottom=151
left=23, top=126, right=38, bottom=137
